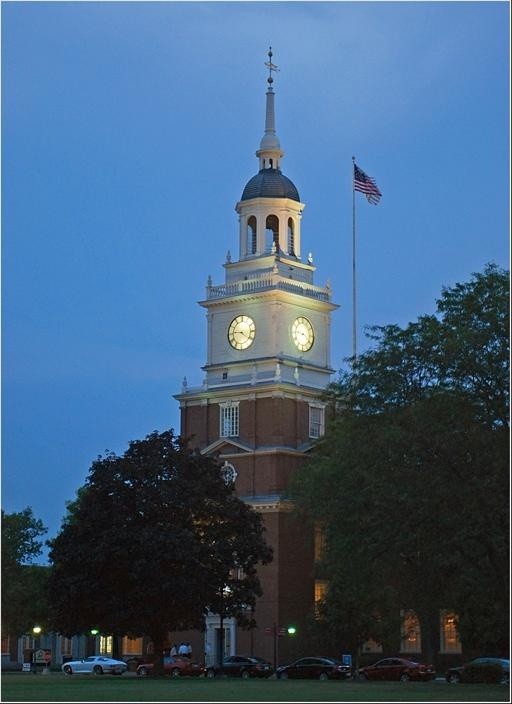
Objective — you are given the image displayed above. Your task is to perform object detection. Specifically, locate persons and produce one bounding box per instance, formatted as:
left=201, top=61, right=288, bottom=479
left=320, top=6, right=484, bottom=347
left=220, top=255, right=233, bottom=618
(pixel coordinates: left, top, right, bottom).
left=171, top=642, right=192, bottom=658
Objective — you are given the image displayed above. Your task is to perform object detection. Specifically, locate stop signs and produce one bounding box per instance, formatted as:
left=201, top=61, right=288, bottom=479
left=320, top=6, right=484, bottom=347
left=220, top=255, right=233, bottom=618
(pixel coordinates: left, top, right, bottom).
left=43, top=651, right=52, bottom=663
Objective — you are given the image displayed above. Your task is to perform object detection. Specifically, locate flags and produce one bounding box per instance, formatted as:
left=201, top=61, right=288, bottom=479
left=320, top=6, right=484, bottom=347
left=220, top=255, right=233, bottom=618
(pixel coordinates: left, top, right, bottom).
left=355, top=162, right=381, bottom=206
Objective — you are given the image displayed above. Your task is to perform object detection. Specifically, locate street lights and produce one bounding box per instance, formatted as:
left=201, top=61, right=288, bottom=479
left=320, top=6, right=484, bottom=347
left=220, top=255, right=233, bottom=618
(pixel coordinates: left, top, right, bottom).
left=33, top=622, right=42, bottom=673
left=91, top=628, right=100, bottom=656
left=264, top=622, right=286, bottom=668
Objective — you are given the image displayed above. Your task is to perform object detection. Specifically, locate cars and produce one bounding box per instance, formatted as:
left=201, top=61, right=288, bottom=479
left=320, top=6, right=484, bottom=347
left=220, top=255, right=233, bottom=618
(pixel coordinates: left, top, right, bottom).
left=354, top=656, right=437, bottom=682
left=202, top=653, right=273, bottom=680
left=275, top=655, right=352, bottom=681
left=136, top=655, right=204, bottom=677
left=445, top=655, right=509, bottom=685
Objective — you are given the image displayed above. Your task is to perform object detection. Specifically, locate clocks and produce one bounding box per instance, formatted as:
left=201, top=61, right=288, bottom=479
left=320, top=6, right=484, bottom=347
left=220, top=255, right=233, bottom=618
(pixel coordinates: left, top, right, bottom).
left=290, top=315, right=315, bottom=352
left=227, top=314, right=256, bottom=351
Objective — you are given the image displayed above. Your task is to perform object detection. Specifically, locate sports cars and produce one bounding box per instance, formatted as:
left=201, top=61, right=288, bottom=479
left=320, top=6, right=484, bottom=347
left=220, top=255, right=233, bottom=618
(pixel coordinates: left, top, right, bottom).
left=62, top=654, right=128, bottom=676
